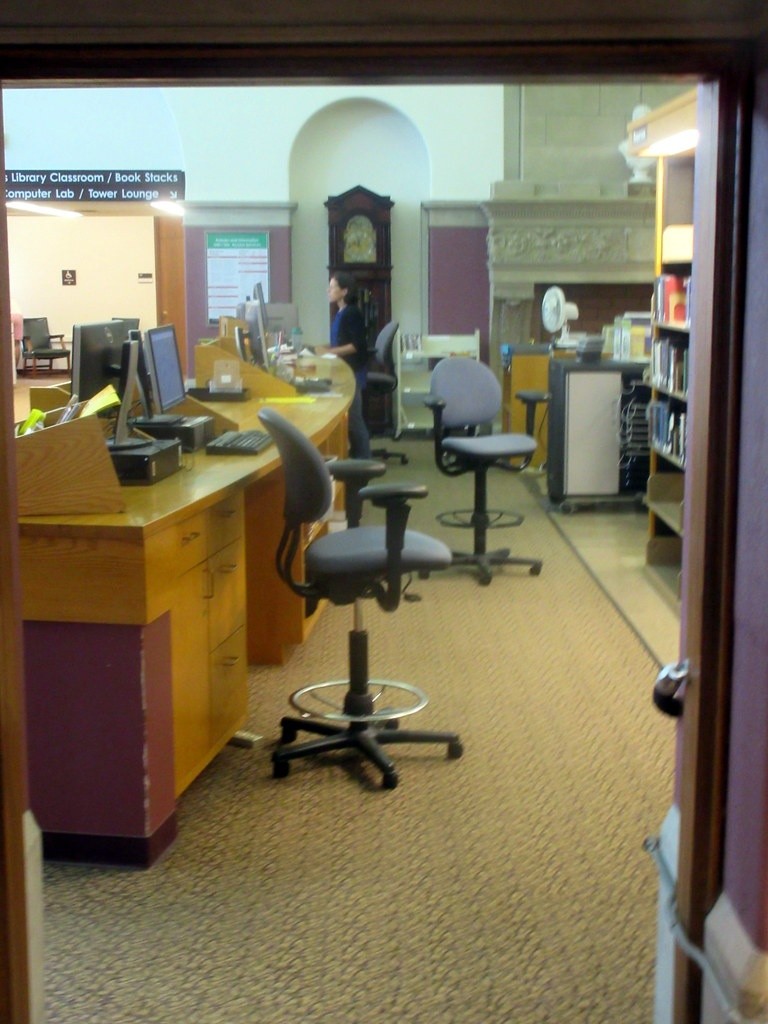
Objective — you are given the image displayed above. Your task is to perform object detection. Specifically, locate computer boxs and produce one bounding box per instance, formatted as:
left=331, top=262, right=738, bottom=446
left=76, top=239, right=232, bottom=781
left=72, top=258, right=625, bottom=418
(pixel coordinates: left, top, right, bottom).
left=131, top=416, right=218, bottom=454
left=107, top=439, right=183, bottom=486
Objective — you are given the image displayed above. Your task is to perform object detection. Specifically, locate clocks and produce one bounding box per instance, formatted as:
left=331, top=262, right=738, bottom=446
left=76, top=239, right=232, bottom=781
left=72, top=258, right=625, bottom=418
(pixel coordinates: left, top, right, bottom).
left=324, top=184, right=395, bottom=436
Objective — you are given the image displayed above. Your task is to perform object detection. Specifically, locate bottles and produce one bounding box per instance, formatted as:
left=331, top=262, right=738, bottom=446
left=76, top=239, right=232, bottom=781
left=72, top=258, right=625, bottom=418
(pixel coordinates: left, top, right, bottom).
left=291, top=326, right=303, bottom=353
left=245, top=296, right=251, bottom=319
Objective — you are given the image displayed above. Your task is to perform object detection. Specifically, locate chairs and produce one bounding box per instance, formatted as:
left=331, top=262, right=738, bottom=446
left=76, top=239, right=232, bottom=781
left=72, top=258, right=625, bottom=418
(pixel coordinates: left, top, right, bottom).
left=20, top=317, right=70, bottom=378
left=360, top=321, right=410, bottom=464
left=257, top=407, right=462, bottom=787
left=420, top=355, right=552, bottom=585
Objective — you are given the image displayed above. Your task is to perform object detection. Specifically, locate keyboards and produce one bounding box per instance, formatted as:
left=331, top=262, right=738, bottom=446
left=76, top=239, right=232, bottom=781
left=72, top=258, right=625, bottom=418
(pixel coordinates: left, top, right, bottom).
left=206, top=432, right=274, bottom=455
left=295, top=380, right=330, bottom=394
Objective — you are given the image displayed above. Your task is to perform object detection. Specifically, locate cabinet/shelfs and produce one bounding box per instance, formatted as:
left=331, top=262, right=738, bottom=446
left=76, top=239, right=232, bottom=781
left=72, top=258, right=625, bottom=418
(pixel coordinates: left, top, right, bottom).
left=12, top=317, right=360, bottom=872
left=643, top=258, right=692, bottom=616
left=627, top=92, right=701, bottom=544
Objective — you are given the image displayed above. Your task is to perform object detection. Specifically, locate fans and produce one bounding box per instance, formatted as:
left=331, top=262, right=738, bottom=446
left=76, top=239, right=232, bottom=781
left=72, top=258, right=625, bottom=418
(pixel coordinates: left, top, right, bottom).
left=542, top=285, right=579, bottom=348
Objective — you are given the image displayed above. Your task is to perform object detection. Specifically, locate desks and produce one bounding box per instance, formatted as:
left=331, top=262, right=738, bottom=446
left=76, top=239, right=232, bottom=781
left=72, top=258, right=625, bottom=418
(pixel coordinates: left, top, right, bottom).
left=552, top=358, right=652, bottom=514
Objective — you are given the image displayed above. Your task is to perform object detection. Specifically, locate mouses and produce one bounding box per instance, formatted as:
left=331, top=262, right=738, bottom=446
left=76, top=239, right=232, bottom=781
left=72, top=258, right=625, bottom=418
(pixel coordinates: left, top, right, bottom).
left=318, top=378, right=332, bottom=385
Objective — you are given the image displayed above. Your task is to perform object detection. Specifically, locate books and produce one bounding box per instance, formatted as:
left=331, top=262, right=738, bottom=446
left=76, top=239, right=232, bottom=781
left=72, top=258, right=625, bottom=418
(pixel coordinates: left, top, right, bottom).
left=654, top=272, right=692, bottom=325
left=649, top=402, right=686, bottom=467
left=647, top=337, right=689, bottom=390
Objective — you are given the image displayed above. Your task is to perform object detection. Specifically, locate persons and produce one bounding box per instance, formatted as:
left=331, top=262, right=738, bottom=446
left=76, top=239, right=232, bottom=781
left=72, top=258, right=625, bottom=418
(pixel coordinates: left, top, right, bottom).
left=327, top=271, right=371, bottom=462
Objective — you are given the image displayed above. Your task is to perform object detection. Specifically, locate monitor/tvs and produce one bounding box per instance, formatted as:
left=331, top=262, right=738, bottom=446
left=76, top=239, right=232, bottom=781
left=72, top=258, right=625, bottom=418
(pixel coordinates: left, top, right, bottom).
left=234, top=283, right=270, bottom=374
left=70, top=318, right=186, bottom=450
